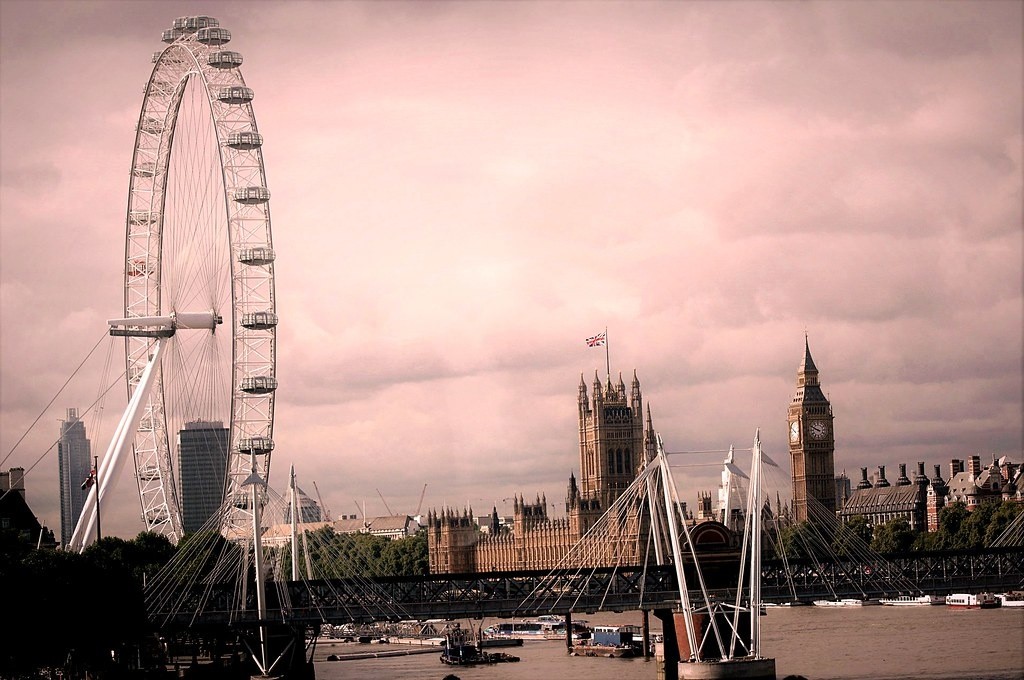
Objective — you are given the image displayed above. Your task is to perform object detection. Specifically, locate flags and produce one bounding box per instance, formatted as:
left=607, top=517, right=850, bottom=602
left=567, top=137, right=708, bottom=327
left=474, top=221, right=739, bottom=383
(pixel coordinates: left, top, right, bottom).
left=584, top=330, right=605, bottom=348
left=81, top=469, right=96, bottom=491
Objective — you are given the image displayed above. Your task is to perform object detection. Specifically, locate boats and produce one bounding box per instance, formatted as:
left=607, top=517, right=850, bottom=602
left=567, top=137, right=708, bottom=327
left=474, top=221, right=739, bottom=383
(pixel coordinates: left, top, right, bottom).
left=812, top=598, right=863, bottom=606
left=993, top=594, right=1024, bottom=607
left=484, top=615, right=592, bottom=640
left=569, top=625, right=655, bottom=658
left=945, top=592, right=995, bottom=609
left=878, top=594, right=945, bottom=606
left=439, top=632, right=521, bottom=666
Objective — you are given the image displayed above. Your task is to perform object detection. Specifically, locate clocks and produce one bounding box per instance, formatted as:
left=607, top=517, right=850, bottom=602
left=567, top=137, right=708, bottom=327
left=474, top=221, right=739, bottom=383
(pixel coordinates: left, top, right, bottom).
left=808, top=419, right=828, bottom=441
left=790, top=420, right=799, bottom=442
